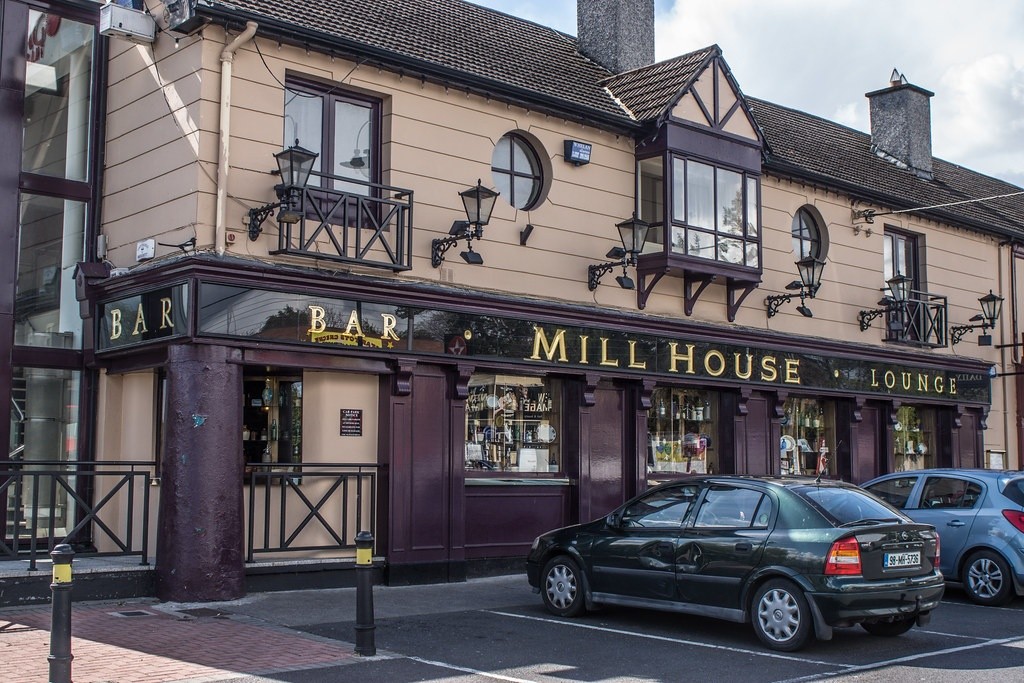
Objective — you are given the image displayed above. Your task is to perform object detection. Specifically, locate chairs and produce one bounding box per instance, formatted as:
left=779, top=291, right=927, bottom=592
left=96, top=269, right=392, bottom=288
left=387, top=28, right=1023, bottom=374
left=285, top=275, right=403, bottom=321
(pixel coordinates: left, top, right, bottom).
left=704, top=494, right=740, bottom=518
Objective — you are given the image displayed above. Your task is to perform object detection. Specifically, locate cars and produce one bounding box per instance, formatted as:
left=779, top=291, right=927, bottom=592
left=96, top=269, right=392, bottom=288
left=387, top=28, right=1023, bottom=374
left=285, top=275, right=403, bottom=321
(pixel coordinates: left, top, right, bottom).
left=523, top=475, right=944, bottom=654
left=855, top=469, right=1024, bottom=607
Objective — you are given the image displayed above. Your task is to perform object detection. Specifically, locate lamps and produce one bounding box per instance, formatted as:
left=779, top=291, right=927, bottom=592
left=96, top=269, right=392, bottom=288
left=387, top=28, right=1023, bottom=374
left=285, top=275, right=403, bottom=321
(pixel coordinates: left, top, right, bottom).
left=276, top=209, right=305, bottom=224
left=448, top=220, right=470, bottom=236
left=861, top=269, right=916, bottom=332
left=952, top=290, right=1004, bottom=345
left=248, top=138, right=320, bottom=241
left=588, top=211, right=651, bottom=290
left=607, top=247, right=626, bottom=258
left=978, top=329, right=992, bottom=346
left=785, top=281, right=803, bottom=291
left=969, top=315, right=985, bottom=324
left=461, top=240, right=484, bottom=265
left=432, top=179, right=501, bottom=268
left=796, top=300, right=813, bottom=318
left=615, top=266, right=636, bottom=290
left=767, top=252, right=827, bottom=318
left=887, top=313, right=903, bottom=331
left=273, top=183, right=289, bottom=201
left=877, top=296, right=895, bottom=306
